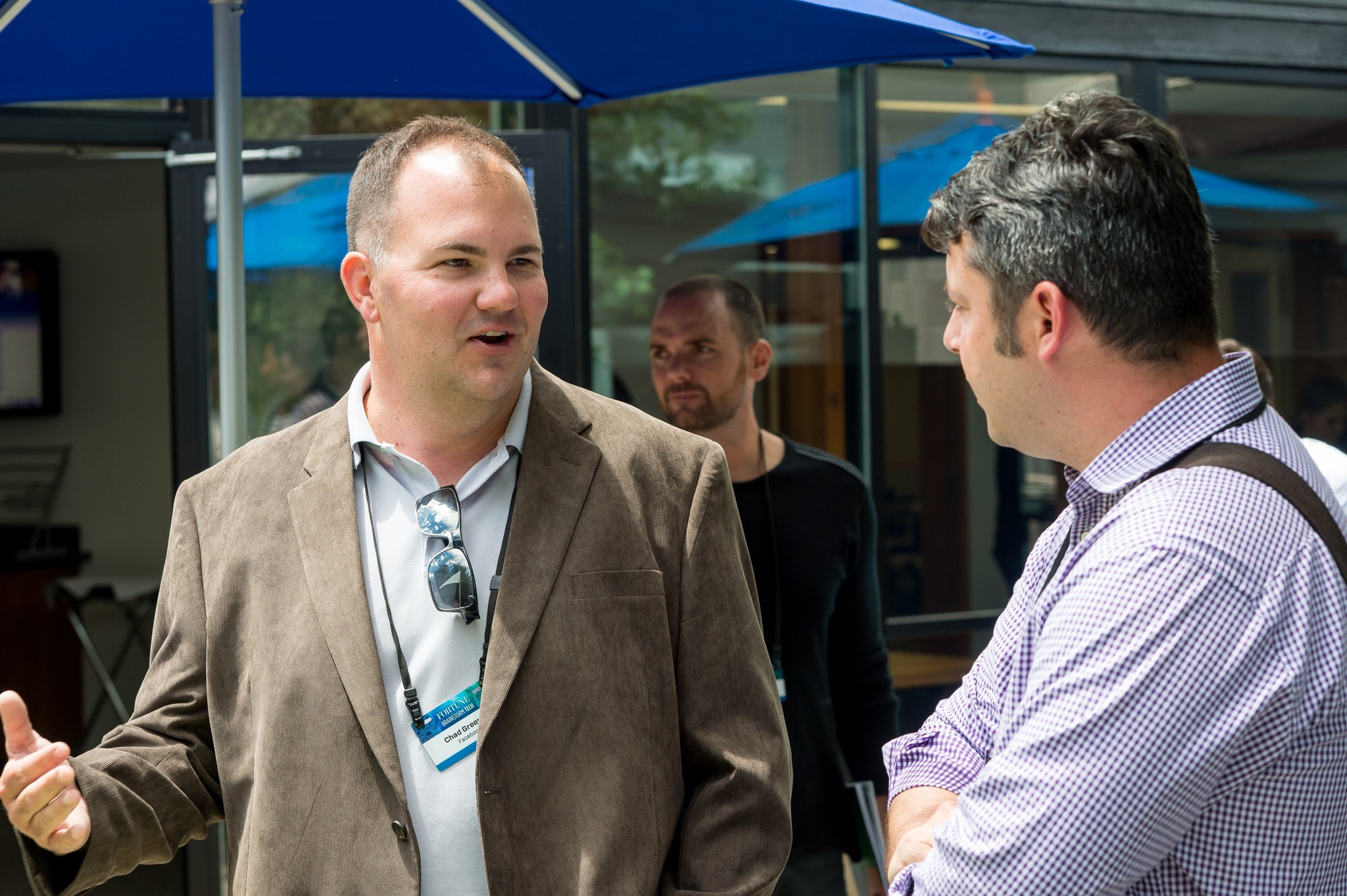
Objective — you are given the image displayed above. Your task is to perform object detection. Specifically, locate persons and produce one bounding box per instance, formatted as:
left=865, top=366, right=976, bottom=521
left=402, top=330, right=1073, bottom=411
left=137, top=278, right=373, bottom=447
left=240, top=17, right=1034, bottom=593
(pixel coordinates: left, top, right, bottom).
left=877, top=87, right=1347, bottom=896
left=0, top=113, right=794, bottom=896
left=638, top=278, right=907, bottom=896
left=254, top=296, right=371, bottom=433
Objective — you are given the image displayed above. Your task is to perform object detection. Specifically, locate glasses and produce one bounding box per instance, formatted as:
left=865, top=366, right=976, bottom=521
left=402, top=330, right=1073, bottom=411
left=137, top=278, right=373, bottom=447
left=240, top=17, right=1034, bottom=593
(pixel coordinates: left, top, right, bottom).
left=416, top=483, right=482, bottom=626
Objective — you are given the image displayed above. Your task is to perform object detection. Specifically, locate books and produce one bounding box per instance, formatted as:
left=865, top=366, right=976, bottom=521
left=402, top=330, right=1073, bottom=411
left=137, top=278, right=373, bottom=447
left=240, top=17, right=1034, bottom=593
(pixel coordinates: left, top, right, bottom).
left=844, top=780, right=892, bottom=893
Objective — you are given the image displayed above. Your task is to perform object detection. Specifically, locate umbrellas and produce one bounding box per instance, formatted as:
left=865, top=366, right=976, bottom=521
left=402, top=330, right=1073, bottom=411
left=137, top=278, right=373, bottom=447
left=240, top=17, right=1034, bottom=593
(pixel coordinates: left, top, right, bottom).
left=0, top=0, right=1034, bottom=460
left=202, top=107, right=1325, bottom=271
